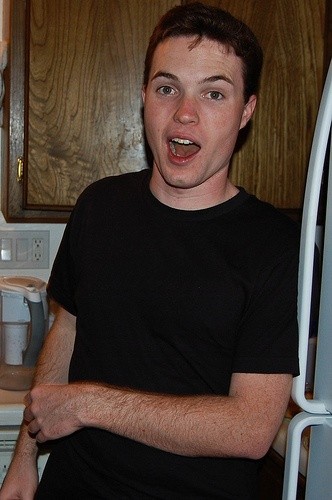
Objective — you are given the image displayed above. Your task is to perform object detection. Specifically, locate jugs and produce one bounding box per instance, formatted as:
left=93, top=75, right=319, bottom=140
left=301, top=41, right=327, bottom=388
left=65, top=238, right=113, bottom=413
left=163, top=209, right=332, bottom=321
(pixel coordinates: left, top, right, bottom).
left=1, top=274, right=52, bottom=391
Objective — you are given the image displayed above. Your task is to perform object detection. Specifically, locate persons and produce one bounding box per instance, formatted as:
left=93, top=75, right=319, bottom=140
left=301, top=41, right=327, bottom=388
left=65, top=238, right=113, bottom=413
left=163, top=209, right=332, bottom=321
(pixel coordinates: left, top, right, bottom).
left=0, top=0, right=300, bottom=500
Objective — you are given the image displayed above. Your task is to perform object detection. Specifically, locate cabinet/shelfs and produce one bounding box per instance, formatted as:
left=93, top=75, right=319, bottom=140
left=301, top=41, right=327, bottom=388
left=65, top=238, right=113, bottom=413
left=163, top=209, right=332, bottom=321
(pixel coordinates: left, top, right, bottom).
left=6, top=1, right=331, bottom=227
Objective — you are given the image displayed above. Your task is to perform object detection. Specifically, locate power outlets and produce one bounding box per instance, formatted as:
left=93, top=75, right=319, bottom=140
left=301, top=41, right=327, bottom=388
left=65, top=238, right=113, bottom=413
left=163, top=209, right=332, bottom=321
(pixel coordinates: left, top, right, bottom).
left=31, top=237, right=44, bottom=263
